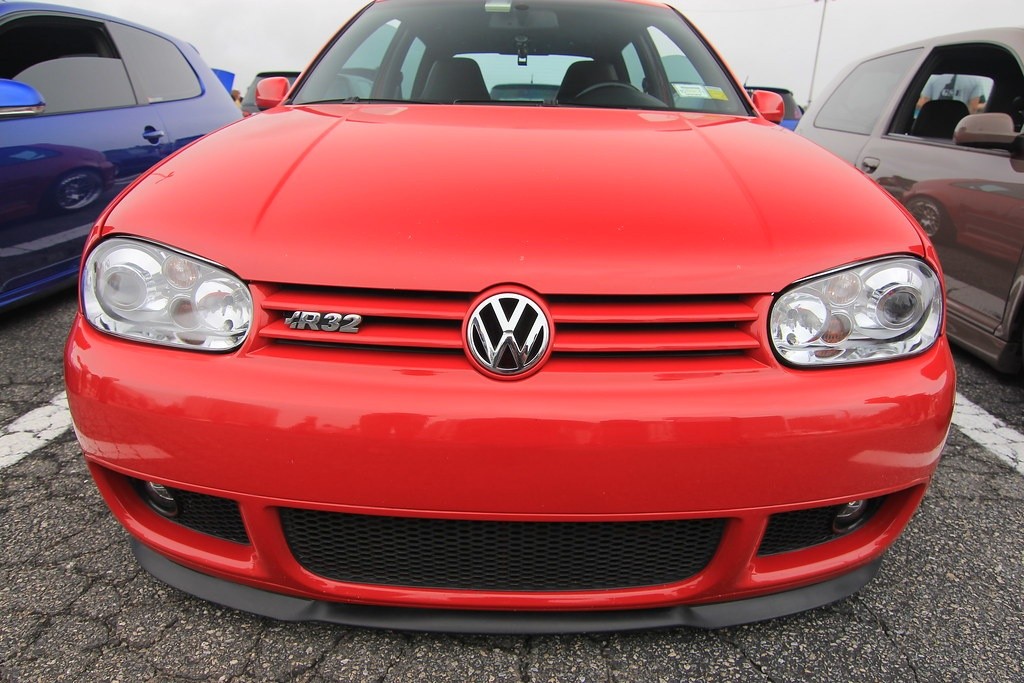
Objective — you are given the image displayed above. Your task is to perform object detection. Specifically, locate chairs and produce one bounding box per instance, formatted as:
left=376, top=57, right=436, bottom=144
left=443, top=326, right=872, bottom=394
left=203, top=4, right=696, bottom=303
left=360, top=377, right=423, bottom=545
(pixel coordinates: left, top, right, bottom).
left=557, top=61, right=621, bottom=102
left=912, top=100, right=970, bottom=139
left=422, top=57, right=491, bottom=100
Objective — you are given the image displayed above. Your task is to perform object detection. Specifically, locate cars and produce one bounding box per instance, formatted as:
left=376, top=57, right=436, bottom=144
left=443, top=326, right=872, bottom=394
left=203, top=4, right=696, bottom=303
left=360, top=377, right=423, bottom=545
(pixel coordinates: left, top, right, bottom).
left=64, top=0, right=956, bottom=633
left=794, top=26, right=1024, bottom=376
left=742, top=86, right=804, bottom=133
left=0, top=3, right=246, bottom=317
left=242, top=70, right=372, bottom=119
